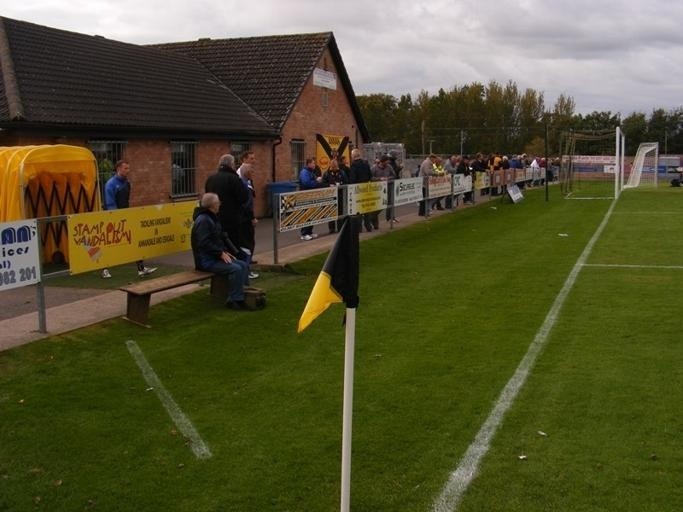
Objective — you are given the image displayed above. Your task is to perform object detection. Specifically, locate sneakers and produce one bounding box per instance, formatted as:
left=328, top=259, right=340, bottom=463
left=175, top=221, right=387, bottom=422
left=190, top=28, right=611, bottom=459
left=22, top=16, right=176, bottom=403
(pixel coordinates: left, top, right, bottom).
left=100, top=268, right=111, bottom=278
left=237, top=301, right=251, bottom=311
left=247, top=271, right=258, bottom=280
left=300, top=233, right=318, bottom=239
left=138, top=266, right=158, bottom=278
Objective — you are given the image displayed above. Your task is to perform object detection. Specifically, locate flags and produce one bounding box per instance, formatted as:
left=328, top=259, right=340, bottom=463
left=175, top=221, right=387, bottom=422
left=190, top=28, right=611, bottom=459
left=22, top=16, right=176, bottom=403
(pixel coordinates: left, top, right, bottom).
left=297, top=217, right=348, bottom=334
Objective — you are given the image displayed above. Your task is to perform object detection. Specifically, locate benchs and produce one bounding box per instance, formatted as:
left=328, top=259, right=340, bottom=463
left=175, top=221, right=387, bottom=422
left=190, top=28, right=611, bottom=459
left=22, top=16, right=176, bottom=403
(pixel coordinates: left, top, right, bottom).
left=118, top=272, right=228, bottom=328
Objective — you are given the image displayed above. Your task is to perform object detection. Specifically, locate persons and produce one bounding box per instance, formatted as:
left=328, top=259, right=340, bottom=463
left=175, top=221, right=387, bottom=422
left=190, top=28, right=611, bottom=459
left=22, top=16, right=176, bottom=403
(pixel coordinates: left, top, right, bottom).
left=191, top=150, right=259, bottom=305
left=298, top=148, right=561, bottom=240
left=99, top=160, right=158, bottom=278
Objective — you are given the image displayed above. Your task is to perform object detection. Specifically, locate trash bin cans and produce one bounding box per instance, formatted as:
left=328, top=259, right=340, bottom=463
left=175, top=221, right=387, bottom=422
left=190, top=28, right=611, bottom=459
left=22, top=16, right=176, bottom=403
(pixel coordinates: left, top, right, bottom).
left=271, top=182, right=297, bottom=219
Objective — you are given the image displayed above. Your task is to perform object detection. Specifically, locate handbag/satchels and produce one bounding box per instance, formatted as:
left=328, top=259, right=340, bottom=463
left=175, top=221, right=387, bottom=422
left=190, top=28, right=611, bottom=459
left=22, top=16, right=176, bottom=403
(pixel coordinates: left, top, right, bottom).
left=225, top=285, right=266, bottom=311
